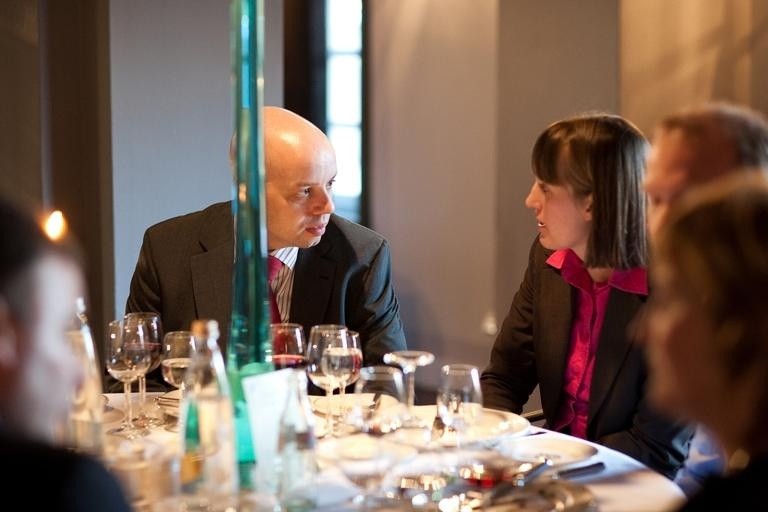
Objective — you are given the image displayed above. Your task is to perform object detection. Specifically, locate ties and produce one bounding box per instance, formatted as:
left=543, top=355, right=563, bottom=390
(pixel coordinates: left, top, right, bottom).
left=269, top=256, right=284, bottom=355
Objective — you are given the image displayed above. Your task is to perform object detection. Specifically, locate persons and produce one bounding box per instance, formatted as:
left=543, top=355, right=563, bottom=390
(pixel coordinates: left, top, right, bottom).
left=627, top=161, right=767, bottom=512
left=104, top=106, right=408, bottom=394
left=633, top=102, right=767, bottom=498
left=1, top=202, right=132, bottom=510
left=479, top=115, right=695, bottom=479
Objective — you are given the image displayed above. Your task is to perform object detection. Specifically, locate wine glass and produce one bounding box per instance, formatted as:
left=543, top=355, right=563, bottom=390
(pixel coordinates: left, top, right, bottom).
left=304, top=324, right=352, bottom=440
left=323, top=331, right=363, bottom=438
left=105, top=319, right=151, bottom=439
left=161, top=332, right=195, bottom=434
left=267, top=321, right=310, bottom=371
left=124, top=311, right=165, bottom=428
left=435, top=362, right=485, bottom=454
left=333, top=409, right=404, bottom=512
left=383, top=350, right=434, bottom=446
left=349, top=366, right=409, bottom=441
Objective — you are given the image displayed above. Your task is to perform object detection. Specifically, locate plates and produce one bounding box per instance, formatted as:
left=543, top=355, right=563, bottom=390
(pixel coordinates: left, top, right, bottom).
left=482, top=409, right=532, bottom=443
left=509, top=437, right=599, bottom=470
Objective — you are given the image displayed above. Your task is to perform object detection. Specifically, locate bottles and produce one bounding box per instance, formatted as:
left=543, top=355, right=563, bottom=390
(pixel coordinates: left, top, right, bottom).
left=176, top=317, right=240, bottom=512
left=63, top=297, right=106, bottom=458
left=274, top=371, right=321, bottom=512
